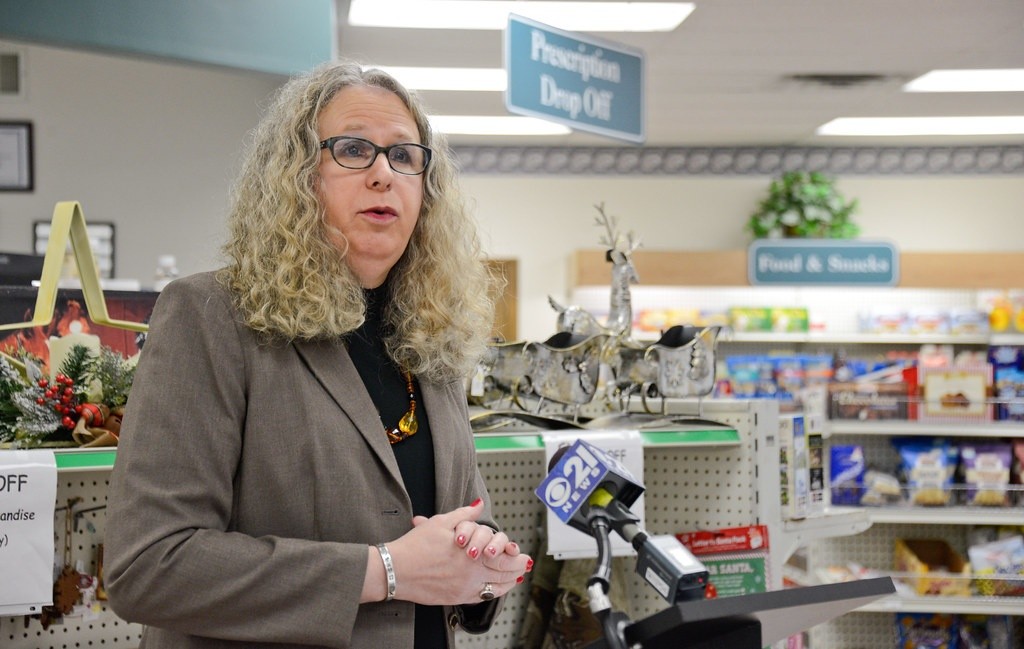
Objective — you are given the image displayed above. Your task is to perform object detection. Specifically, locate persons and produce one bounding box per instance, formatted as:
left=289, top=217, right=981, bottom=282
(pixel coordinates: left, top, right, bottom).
left=106, top=69, right=535, bottom=649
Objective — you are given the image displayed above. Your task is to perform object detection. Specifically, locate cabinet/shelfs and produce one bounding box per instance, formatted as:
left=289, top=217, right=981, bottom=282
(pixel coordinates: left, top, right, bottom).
left=603, top=328, right=1024, bottom=649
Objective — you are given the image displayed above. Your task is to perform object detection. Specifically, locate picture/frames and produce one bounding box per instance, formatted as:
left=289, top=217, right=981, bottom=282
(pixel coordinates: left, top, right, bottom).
left=0, top=119, right=34, bottom=194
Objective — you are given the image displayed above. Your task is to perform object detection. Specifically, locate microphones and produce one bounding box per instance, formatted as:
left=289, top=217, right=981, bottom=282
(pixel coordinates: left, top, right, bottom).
left=535, top=439, right=709, bottom=606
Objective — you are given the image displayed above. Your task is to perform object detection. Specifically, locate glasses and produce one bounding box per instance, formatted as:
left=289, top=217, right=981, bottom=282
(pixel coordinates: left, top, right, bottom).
left=318, top=135, right=432, bottom=175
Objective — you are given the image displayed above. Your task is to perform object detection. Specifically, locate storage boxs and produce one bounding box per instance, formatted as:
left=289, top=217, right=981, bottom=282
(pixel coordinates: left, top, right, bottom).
left=891, top=536, right=972, bottom=597
left=778, top=411, right=831, bottom=523
left=916, top=359, right=1000, bottom=422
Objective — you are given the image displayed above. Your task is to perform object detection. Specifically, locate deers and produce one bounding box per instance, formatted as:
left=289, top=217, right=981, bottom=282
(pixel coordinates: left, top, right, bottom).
left=547, top=200, right=645, bottom=404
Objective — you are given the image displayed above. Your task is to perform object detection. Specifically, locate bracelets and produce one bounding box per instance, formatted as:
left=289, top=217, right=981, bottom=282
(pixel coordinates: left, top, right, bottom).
left=373, top=542, right=396, bottom=602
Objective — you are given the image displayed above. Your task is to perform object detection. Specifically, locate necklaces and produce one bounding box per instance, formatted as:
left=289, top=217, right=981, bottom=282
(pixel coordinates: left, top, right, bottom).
left=387, top=356, right=417, bottom=446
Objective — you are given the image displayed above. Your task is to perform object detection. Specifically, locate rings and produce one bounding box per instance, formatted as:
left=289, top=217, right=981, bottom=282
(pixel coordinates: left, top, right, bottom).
left=480, top=582, right=494, bottom=601
